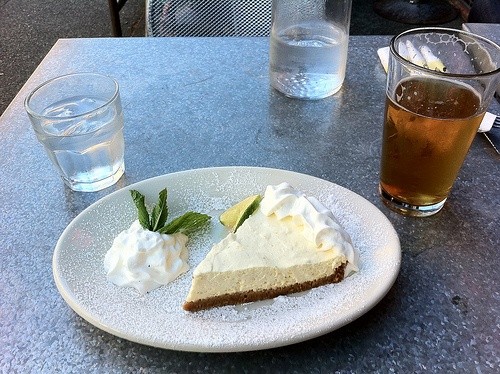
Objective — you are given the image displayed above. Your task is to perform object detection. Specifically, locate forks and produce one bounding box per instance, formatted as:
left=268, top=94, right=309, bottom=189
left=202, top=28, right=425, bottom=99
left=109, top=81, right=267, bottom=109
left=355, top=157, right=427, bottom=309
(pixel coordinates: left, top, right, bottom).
left=493, top=116, right=500, bottom=128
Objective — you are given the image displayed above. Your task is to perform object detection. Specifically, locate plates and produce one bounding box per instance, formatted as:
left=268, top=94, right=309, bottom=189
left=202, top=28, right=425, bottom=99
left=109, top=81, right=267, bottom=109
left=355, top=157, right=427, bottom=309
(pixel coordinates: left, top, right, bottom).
left=52, top=165, right=400, bottom=353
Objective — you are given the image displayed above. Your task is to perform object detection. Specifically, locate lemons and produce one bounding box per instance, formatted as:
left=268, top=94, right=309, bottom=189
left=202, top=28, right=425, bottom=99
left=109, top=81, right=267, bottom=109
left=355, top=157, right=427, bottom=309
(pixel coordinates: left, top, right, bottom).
left=232, top=194, right=260, bottom=233
left=220, top=195, right=258, bottom=226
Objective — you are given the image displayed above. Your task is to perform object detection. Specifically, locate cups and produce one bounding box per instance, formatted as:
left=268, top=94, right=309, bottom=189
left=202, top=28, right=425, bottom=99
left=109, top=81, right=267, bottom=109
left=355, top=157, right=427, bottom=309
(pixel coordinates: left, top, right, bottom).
left=24, top=72, right=125, bottom=193
left=269, top=0, right=352, bottom=101
left=379, top=26, right=500, bottom=218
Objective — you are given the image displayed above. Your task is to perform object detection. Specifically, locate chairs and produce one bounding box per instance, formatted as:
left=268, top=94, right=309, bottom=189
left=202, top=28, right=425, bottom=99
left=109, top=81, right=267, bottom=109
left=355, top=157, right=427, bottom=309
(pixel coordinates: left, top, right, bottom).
left=145, top=0, right=326, bottom=38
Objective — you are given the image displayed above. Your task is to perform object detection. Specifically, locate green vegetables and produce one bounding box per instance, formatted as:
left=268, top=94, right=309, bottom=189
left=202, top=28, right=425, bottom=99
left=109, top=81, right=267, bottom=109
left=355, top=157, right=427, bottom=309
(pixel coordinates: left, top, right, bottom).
left=129, top=187, right=212, bottom=235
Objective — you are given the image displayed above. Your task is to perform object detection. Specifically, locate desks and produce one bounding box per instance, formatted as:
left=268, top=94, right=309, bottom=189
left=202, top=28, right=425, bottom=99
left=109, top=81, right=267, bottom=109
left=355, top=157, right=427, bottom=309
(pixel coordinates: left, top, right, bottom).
left=0, top=23, right=500, bottom=374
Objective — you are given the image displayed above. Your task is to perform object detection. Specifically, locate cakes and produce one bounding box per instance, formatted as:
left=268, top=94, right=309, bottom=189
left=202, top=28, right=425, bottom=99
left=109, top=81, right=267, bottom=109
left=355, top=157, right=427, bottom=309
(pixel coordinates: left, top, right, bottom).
left=182, top=182, right=359, bottom=312
